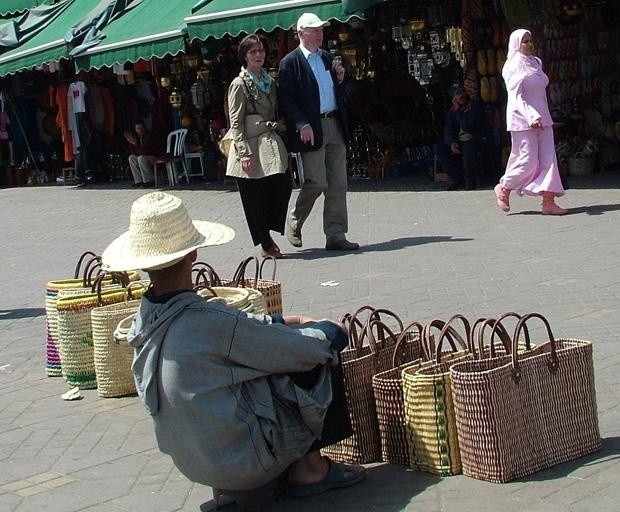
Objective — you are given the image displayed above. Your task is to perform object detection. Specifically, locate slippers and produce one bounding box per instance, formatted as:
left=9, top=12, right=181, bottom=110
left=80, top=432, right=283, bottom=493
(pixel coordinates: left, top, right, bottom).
left=284, top=457, right=369, bottom=495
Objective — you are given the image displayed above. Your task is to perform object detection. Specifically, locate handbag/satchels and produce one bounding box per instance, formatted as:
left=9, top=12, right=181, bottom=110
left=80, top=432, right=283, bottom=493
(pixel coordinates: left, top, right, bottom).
left=190, top=262, right=221, bottom=302
left=403, top=314, right=537, bottom=477
left=220, top=255, right=283, bottom=317
left=57, top=271, right=151, bottom=390
left=58, top=263, right=150, bottom=297
left=45, top=252, right=141, bottom=377
left=321, top=310, right=435, bottom=465
left=372, top=322, right=458, bottom=466
left=450, top=312, right=601, bottom=484
left=91, top=281, right=147, bottom=398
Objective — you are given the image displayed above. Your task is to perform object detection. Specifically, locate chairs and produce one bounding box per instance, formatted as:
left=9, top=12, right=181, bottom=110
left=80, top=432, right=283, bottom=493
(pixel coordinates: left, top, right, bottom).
left=289, top=151, right=304, bottom=186
left=149, top=127, right=190, bottom=187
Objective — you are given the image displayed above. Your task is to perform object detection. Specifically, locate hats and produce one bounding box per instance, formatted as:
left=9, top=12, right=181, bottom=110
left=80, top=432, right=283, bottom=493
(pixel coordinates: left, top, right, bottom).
left=102, top=193, right=235, bottom=273
left=297, top=13, right=331, bottom=32
left=447, top=83, right=464, bottom=96
left=248, top=287, right=268, bottom=315
left=196, top=286, right=256, bottom=315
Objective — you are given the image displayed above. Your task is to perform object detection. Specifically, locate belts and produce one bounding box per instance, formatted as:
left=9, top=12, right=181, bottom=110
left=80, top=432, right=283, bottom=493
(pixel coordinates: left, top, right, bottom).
left=320, top=112, right=338, bottom=118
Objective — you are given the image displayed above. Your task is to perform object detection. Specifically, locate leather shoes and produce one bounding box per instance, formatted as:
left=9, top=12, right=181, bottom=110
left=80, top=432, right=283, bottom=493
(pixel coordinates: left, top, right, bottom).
left=261, top=248, right=281, bottom=257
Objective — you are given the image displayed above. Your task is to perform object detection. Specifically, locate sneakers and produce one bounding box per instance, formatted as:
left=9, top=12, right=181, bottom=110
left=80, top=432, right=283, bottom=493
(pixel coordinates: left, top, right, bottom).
left=494, top=183, right=509, bottom=211
left=1, top=185, right=5, bottom=189
left=542, top=202, right=569, bottom=215
left=78, top=181, right=86, bottom=187
left=143, top=183, right=153, bottom=189
left=132, top=184, right=142, bottom=189
left=88, top=176, right=96, bottom=185
left=287, top=221, right=302, bottom=247
left=465, top=181, right=476, bottom=190
left=325, top=240, right=359, bottom=251
left=446, top=180, right=465, bottom=191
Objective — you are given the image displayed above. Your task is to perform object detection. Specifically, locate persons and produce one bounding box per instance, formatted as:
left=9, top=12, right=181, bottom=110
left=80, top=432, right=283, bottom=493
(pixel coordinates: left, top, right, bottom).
left=494, top=29, right=569, bottom=215
left=220, top=34, right=292, bottom=258
left=175, top=109, right=205, bottom=182
left=125, top=119, right=161, bottom=188
left=435, top=84, right=487, bottom=190
left=101, top=193, right=367, bottom=496
left=279, top=13, right=361, bottom=251
